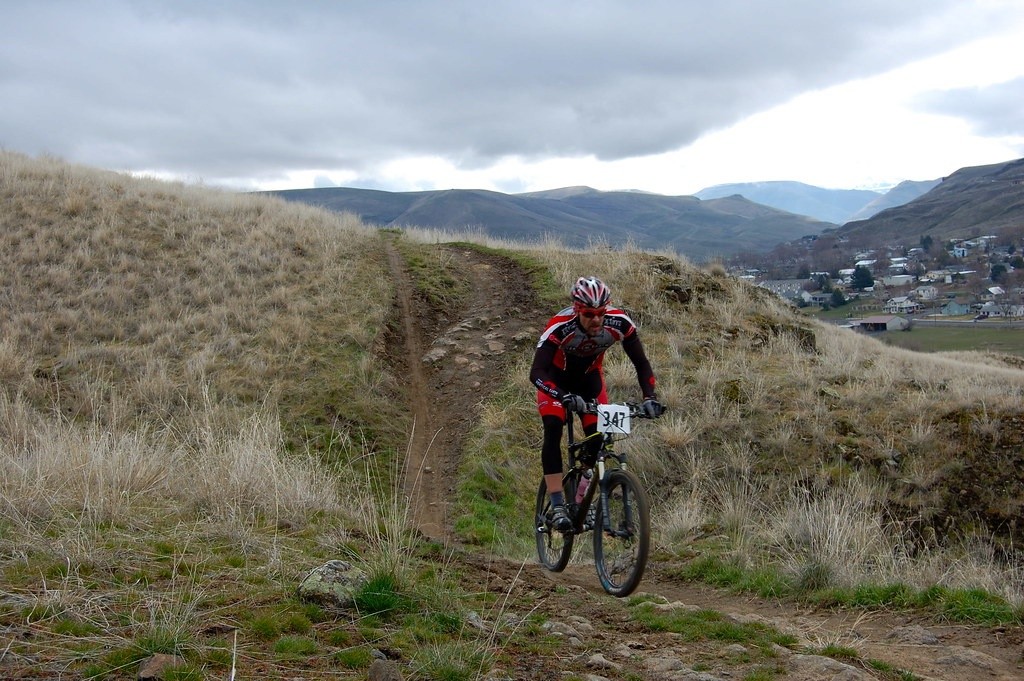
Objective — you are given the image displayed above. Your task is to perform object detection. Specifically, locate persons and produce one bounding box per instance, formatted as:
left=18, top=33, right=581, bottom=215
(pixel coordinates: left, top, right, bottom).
left=529, top=275, right=662, bottom=531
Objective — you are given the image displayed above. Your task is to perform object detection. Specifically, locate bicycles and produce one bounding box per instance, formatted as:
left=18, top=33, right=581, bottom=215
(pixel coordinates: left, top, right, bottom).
left=535, top=399, right=667, bottom=597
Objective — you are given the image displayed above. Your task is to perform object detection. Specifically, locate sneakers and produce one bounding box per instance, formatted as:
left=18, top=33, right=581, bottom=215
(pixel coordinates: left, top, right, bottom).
left=548, top=505, right=571, bottom=530
left=577, top=503, right=596, bottom=527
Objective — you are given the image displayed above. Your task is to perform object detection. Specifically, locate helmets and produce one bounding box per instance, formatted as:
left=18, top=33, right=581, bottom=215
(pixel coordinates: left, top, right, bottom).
left=571, top=278, right=612, bottom=310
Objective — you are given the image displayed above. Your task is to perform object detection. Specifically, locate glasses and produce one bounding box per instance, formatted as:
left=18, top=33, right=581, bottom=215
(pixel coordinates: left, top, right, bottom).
left=577, top=308, right=607, bottom=318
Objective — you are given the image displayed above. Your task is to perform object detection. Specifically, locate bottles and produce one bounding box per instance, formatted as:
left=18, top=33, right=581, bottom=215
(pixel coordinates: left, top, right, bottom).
left=575, top=469, right=592, bottom=504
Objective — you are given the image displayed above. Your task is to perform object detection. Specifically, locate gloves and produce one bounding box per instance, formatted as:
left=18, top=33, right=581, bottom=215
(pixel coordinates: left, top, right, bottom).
left=642, top=400, right=662, bottom=420
left=562, top=395, right=587, bottom=414
